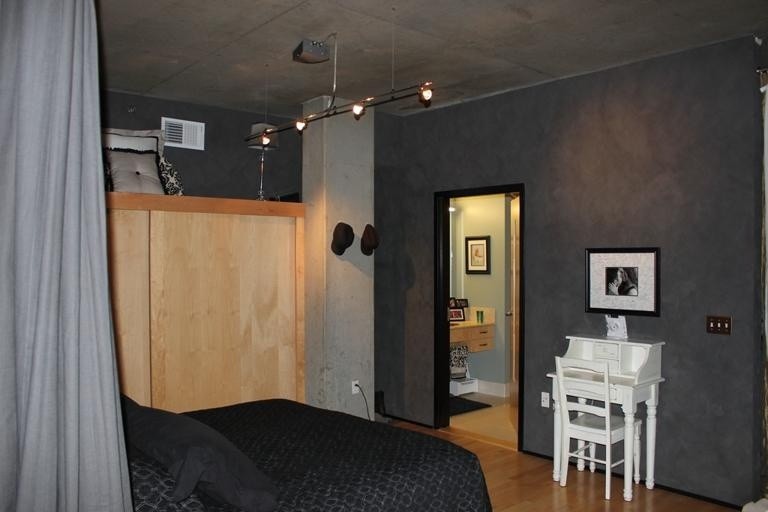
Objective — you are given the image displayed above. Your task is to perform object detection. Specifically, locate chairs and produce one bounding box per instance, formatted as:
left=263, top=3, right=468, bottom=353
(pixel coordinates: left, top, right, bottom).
left=554, top=355, right=643, bottom=498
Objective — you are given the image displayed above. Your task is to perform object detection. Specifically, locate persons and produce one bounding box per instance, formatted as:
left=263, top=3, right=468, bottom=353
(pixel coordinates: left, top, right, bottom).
left=608, top=268, right=638, bottom=295
left=607, top=268, right=622, bottom=295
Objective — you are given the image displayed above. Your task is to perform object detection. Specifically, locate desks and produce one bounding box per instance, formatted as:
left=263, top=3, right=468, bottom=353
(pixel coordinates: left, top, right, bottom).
left=544, top=332, right=666, bottom=492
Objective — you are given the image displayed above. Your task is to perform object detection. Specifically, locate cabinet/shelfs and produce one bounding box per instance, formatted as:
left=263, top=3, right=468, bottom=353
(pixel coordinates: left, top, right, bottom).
left=449, top=325, right=496, bottom=353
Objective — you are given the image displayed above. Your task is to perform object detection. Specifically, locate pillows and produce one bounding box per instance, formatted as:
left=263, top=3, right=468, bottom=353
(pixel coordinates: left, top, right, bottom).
left=103, top=129, right=166, bottom=195
left=121, top=393, right=281, bottom=511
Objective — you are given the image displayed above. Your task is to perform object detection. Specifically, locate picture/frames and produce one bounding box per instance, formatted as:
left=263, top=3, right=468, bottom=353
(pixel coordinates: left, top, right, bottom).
left=449, top=296, right=469, bottom=321
left=585, top=247, right=660, bottom=319
left=465, top=235, right=491, bottom=276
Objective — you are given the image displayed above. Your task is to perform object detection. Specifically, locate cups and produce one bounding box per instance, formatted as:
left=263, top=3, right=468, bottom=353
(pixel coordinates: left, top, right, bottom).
left=476, top=311, right=485, bottom=324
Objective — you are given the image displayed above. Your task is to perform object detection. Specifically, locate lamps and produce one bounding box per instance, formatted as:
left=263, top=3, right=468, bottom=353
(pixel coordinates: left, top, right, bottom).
left=247, top=122, right=282, bottom=202
left=258, top=86, right=433, bottom=148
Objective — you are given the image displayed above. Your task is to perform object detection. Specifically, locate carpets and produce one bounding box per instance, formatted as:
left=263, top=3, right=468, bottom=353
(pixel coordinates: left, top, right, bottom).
left=450, top=396, right=493, bottom=418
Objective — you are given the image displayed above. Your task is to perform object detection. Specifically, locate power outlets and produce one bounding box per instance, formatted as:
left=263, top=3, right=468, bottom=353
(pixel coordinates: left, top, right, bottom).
left=352, top=380, right=360, bottom=394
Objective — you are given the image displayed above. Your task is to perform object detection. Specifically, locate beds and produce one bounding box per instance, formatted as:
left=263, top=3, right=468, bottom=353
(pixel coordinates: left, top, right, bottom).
left=125, top=399, right=478, bottom=511
